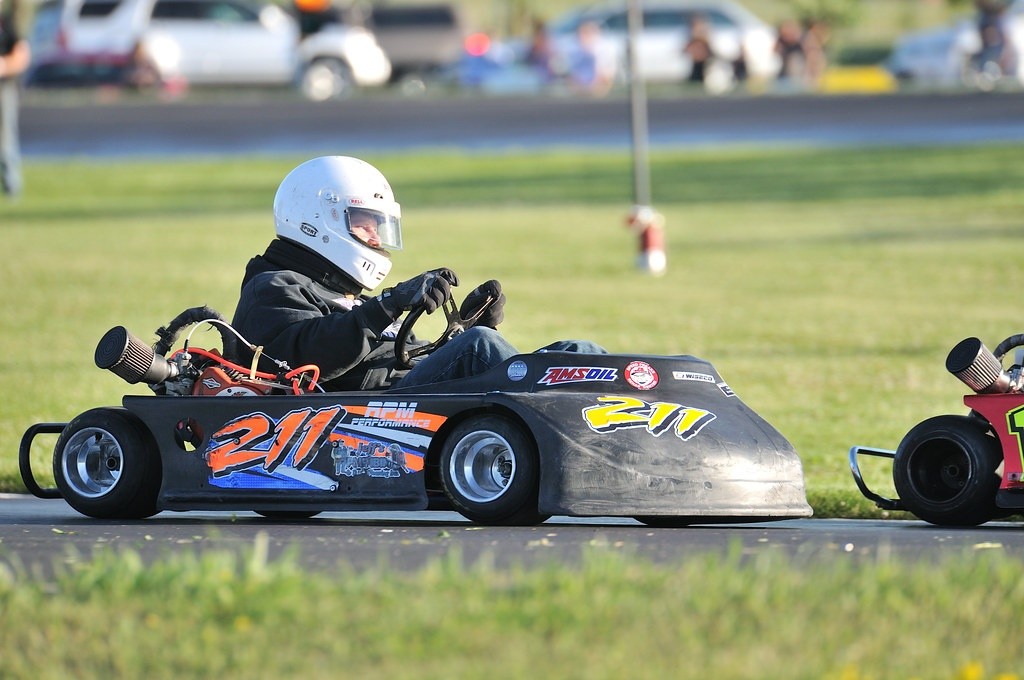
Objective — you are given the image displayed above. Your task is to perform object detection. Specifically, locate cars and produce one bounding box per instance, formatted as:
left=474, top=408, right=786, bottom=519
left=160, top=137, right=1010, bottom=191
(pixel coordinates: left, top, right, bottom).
left=29, top=0, right=393, bottom=103
left=301, top=0, right=783, bottom=101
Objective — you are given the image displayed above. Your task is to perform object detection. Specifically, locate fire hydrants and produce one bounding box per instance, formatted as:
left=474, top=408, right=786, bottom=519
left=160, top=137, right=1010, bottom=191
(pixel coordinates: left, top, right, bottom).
left=624, top=206, right=669, bottom=276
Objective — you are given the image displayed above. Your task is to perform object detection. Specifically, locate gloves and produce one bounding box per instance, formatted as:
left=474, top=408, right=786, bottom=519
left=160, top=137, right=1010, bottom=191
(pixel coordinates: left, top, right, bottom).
left=382, top=267, right=460, bottom=315
left=460, top=280, right=506, bottom=328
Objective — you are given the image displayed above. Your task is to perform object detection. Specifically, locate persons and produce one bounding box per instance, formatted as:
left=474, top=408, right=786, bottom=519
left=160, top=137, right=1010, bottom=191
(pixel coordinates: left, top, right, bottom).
left=681, top=18, right=721, bottom=82
left=233, top=154, right=506, bottom=394
left=770, top=15, right=809, bottom=80
left=553, top=19, right=618, bottom=100
left=727, top=43, right=752, bottom=89
left=1, top=2, right=35, bottom=205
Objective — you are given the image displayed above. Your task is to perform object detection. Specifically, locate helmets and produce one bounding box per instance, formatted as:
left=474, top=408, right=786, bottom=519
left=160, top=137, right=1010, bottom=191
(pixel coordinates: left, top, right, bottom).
left=273, top=156, right=402, bottom=291
